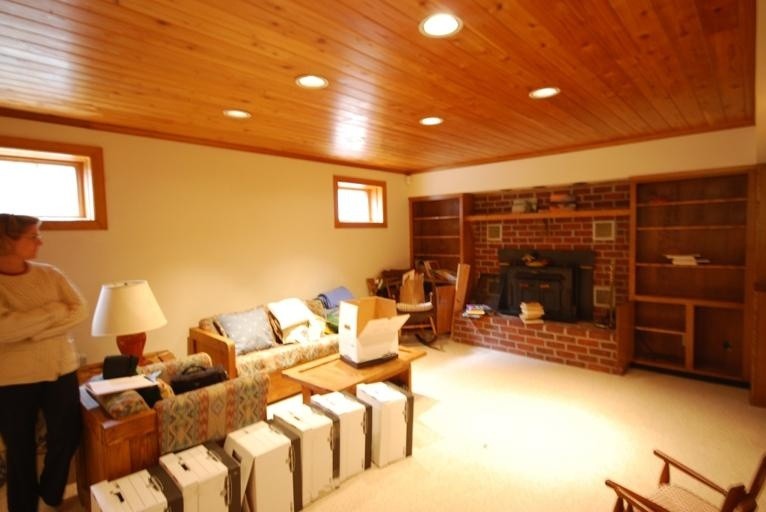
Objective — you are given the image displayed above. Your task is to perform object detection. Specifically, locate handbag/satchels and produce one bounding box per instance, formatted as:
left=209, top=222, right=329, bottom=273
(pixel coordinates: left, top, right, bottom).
left=171, top=365, right=227, bottom=393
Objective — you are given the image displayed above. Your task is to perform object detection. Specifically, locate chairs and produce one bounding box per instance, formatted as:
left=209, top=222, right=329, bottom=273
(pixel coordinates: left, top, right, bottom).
left=386, top=278, right=439, bottom=345
left=77, top=351, right=269, bottom=512
left=606, top=450, right=766, bottom=512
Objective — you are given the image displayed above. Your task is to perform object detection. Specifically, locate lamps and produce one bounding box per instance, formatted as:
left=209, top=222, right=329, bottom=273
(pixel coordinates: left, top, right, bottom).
left=91, top=279, right=167, bottom=366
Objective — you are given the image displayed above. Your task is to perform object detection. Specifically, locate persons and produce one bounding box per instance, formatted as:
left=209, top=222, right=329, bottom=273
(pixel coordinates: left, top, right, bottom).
left=0, top=214, right=90, bottom=512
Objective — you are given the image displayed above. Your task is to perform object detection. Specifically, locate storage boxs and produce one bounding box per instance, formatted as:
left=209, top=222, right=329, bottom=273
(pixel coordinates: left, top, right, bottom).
left=274, top=402, right=340, bottom=512
left=159, top=440, right=241, bottom=511
left=223, top=420, right=302, bottom=512
left=356, top=380, right=414, bottom=467
left=89, top=464, right=183, bottom=512
left=310, top=389, right=372, bottom=489
left=339, top=297, right=411, bottom=370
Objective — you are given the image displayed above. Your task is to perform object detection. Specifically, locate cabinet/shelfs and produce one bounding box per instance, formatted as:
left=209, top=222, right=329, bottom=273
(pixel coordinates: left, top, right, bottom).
left=615, top=163, right=765, bottom=407
left=409, top=192, right=474, bottom=335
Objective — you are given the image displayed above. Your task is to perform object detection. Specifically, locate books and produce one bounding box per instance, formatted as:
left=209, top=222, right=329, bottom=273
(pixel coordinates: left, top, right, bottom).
left=461, top=301, right=492, bottom=318
left=664, top=251, right=713, bottom=265
left=518, top=300, right=544, bottom=324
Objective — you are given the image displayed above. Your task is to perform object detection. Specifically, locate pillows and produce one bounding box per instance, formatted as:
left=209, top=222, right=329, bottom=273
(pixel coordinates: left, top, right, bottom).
left=157, top=378, right=174, bottom=401
left=215, top=306, right=278, bottom=356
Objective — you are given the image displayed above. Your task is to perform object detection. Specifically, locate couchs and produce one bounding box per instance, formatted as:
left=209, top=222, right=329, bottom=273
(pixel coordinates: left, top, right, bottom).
left=187, top=286, right=355, bottom=405
